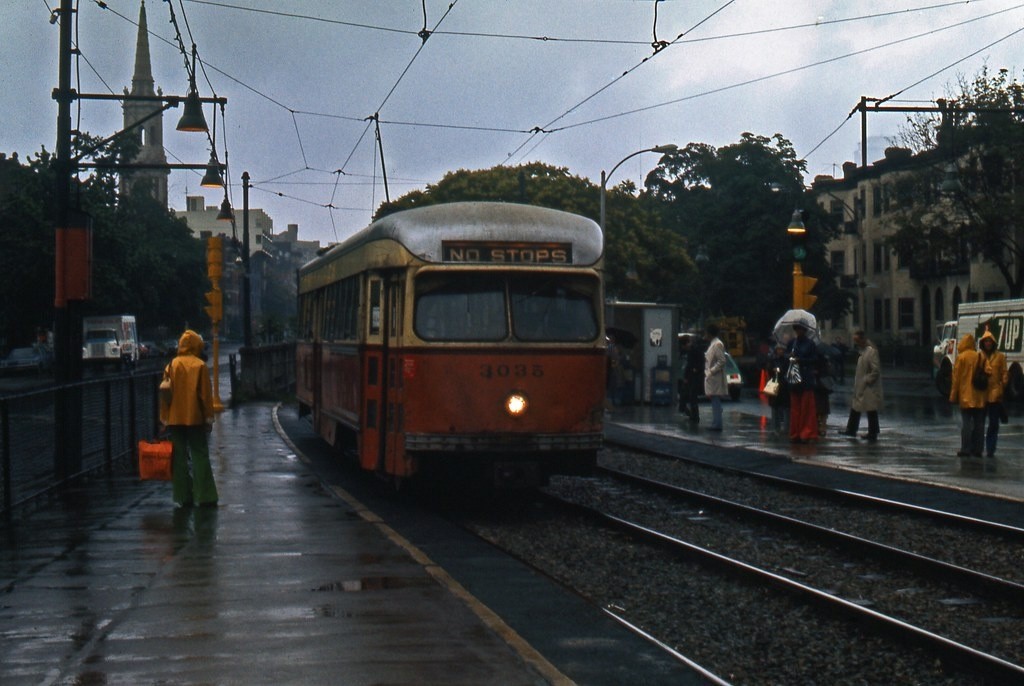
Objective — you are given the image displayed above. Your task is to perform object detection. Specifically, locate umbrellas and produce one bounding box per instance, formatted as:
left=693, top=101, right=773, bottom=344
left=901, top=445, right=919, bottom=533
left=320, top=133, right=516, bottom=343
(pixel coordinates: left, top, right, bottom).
left=604, top=326, right=639, bottom=352
left=775, top=301, right=822, bottom=359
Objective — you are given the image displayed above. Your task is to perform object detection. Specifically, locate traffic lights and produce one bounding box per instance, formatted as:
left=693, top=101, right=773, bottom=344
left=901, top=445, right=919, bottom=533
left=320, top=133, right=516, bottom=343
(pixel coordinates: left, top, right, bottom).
left=204, top=292, right=222, bottom=321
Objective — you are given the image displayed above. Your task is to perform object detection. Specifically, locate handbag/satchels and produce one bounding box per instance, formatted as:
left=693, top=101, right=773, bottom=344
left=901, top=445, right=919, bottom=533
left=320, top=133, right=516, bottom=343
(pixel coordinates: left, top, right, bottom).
left=788, top=361, right=801, bottom=384
left=139, top=428, right=172, bottom=480
left=764, top=373, right=780, bottom=395
left=972, top=353, right=990, bottom=390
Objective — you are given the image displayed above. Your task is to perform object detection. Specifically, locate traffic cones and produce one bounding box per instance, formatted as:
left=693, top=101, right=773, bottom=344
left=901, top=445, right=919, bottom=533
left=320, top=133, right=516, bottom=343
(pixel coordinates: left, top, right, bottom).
left=758, top=369, right=767, bottom=391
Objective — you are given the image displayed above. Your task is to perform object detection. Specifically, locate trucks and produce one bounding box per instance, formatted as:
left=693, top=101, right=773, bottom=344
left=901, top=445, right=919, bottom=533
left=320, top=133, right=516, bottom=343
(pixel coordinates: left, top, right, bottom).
left=931, top=299, right=1024, bottom=401
left=82, top=316, right=140, bottom=367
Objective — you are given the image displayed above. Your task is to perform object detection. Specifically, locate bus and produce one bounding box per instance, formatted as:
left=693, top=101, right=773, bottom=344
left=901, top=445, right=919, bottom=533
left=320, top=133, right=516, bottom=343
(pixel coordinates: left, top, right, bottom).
left=294, top=112, right=609, bottom=490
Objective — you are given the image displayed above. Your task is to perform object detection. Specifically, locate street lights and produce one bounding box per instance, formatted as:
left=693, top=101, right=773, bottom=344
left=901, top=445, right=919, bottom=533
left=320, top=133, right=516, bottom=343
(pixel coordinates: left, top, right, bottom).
left=771, top=182, right=864, bottom=337
left=600, top=143, right=678, bottom=248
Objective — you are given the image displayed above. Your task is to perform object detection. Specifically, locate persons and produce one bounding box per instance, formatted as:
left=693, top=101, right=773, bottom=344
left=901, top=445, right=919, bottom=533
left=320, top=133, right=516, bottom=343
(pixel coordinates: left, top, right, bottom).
left=603, top=332, right=625, bottom=411
left=680, top=335, right=705, bottom=423
left=830, top=336, right=848, bottom=384
left=950, top=331, right=1009, bottom=457
left=767, top=318, right=830, bottom=444
left=838, top=331, right=883, bottom=440
left=705, top=324, right=728, bottom=432
left=159, top=329, right=219, bottom=511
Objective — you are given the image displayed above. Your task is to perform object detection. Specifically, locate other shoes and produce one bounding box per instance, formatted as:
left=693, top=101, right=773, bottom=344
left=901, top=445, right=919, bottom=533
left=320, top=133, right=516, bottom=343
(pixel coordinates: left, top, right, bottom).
left=838, top=430, right=856, bottom=437
left=958, top=450, right=981, bottom=456
left=861, top=433, right=877, bottom=440
left=986, top=451, right=994, bottom=457
left=708, top=427, right=722, bottom=431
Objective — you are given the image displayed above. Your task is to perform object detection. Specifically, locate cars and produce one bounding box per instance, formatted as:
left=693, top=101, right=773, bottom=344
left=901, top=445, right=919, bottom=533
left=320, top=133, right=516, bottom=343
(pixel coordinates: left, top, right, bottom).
left=0, top=347, right=52, bottom=377
left=138, top=338, right=178, bottom=358
left=678, top=350, right=744, bottom=398
